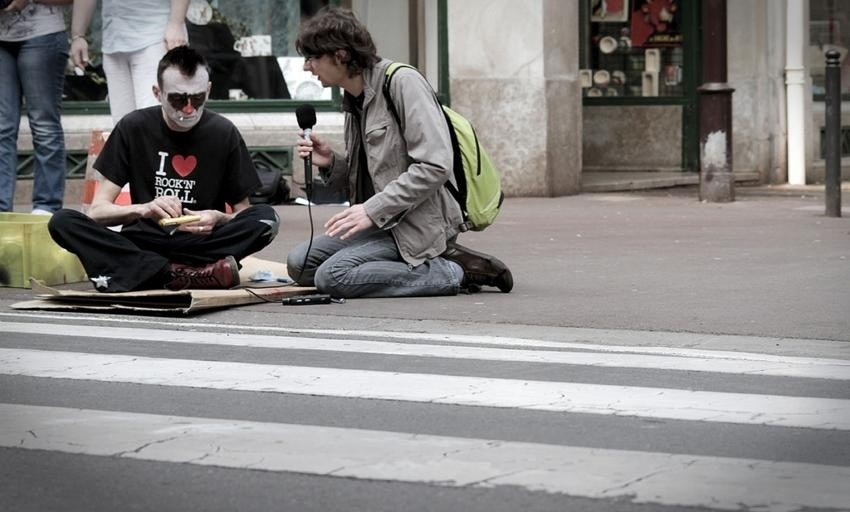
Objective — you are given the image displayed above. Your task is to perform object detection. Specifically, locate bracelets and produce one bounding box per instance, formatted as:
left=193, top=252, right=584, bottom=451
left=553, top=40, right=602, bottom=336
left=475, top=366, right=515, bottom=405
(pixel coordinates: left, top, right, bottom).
left=71, top=34, right=85, bottom=42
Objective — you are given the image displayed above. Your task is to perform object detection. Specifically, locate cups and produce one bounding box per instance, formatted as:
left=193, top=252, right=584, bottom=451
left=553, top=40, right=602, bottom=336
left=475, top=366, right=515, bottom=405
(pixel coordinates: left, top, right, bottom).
left=232, top=34, right=272, bottom=57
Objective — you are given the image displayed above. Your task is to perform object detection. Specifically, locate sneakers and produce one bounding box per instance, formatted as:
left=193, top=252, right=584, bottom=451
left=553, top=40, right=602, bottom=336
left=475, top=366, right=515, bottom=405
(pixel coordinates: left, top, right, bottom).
left=439, top=242, right=513, bottom=293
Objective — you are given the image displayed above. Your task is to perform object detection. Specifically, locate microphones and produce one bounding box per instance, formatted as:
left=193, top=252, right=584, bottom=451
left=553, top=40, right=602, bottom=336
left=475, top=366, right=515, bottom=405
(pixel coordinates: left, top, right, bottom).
left=296, top=104, right=316, bottom=200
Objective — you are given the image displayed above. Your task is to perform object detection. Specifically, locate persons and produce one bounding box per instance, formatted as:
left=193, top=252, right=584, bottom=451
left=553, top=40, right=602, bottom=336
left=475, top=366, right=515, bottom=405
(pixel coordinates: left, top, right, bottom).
left=47, top=45, right=282, bottom=294
left=287, top=6, right=514, bottom=297
left=0, top=1, right=73, bottom=215
left=68, top=1, right=191, bottom=129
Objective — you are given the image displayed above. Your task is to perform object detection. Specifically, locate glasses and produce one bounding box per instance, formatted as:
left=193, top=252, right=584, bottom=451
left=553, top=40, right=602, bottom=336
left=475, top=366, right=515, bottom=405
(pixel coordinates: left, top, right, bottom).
left=305, top=55, right=313, bottom=65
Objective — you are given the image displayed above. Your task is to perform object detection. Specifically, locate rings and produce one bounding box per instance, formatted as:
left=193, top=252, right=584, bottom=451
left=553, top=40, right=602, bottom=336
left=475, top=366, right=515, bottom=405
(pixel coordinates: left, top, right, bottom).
left=199, top=225, right=204, bottom=231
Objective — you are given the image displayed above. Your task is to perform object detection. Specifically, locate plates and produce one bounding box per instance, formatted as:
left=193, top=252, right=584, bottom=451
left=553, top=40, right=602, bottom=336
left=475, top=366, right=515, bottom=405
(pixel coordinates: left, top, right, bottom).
left=186, top=0, right=212, bottom=25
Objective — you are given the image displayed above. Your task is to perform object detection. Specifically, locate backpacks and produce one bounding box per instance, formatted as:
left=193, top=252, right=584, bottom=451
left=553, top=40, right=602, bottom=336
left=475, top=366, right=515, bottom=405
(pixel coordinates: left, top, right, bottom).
left=382, top=62, right=504, bottom=233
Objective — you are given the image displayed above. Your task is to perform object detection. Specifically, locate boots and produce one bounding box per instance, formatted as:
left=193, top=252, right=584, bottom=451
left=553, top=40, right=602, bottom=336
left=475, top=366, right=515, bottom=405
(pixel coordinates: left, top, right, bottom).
left=165, top=255, right=240, bottom=290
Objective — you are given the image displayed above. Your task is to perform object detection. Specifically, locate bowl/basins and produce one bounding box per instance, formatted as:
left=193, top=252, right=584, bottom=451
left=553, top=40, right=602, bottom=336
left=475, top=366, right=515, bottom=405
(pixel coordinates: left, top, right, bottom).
left=594, top=69, right=610, bottom=86
left=600, top=36, right=618, bottom=52
